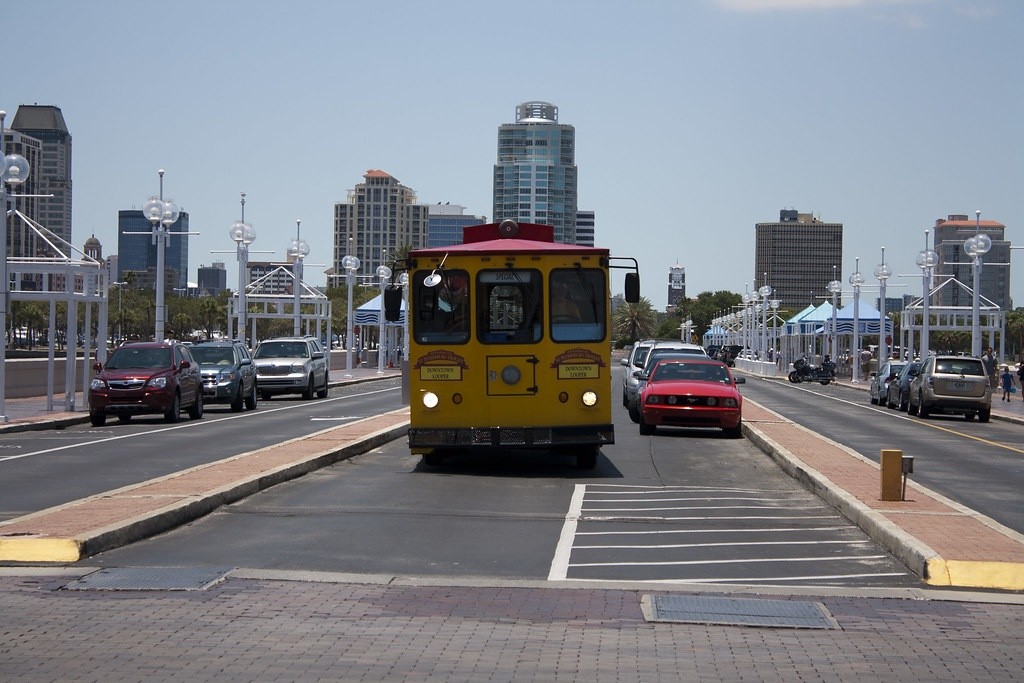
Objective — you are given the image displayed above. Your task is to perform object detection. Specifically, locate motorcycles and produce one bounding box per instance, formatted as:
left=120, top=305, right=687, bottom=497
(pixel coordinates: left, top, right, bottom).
left=788, top=353, right=836, bottom=386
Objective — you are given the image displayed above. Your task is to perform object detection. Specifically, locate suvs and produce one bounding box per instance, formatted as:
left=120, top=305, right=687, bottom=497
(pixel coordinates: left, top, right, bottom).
left=620, top=338, right=721, bottom=421
left=183, top=339, right=257, bottom=412
left=88, top=339, right=205, bottom=426
left=907, top=353, right=992, bottom=422
left=251, top=334, right=329, bottom=400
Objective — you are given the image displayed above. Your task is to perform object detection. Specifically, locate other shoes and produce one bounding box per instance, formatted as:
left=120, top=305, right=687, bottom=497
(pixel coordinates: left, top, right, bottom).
left=1007, top=399, right=1010, bottom=403
left=862, top=378, right=868, bottom=381
left=1002, top=397, right=1005, bottom=401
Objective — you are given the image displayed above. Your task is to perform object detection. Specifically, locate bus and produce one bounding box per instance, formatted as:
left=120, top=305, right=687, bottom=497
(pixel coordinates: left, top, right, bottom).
left=384, top=219, right=640, bottom=455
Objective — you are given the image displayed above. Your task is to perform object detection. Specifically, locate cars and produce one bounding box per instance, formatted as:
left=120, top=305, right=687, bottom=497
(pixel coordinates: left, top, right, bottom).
left=705, top=344, right=758, bottom=366
left=638, top=359, right=745, bottom=435
left=870, top=360, right=924, bottom=411
left=190, top=330, right=228, bottom=340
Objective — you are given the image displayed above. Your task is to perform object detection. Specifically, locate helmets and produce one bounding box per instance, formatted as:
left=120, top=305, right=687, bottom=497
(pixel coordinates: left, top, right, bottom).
left=825, top=354, right=829, bottom=359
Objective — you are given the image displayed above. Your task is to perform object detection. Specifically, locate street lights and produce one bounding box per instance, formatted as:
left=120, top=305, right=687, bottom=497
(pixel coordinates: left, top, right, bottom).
left=711, top=286, right=780, bottom=363
left=0, top=151, right=29, bottom=423
left=873, top=263, right=893, bottom=368
left=145, top=197, right=409, bottom=378
left=849, top=272, right=866, bottom=382
left=826, top=280, right=843, bottom=371
left=916, top=250, right=939, bottom=359
left=964, top=234, right=992, bottom=358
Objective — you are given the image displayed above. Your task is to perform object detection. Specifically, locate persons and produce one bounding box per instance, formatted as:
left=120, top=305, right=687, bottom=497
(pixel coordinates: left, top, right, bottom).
left=1019, top=350, right=1024, bottom=369
left=1017, top=365, right=1024, bottom=401
left=860, top=346, right=871, bottom=381
left=552, top=280, right=575, bottom=313
left=983, top=347, right=997, bottom=392
left=1002, top=367, right=1015, bottom=402
left=905, top=351, right=908, bottom=360
left=767, top=350, right=773, bottom=362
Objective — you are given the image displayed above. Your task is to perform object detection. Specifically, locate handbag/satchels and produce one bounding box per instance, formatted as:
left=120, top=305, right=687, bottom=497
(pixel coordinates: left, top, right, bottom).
left=1011, top=386, right=1016, bottom=393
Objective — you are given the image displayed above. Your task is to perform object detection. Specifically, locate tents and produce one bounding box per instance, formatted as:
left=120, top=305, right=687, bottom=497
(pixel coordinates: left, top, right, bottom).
left=823, top=300, right=893, bottom=361
left=705, top=326, right=728, bottom=347
left=781, top=305, right=823, bottom=372
left=353, top=294, right=409, bottom=348
left=797, top=301, right=843, bottom=374
